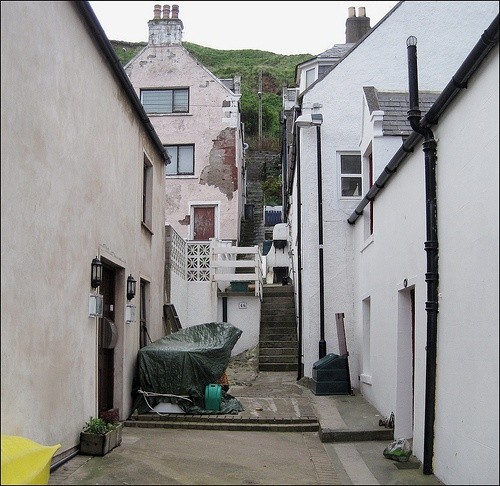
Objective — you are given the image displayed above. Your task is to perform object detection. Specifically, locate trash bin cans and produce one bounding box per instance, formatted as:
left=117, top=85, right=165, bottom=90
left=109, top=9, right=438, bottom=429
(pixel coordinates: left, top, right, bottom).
left=310, top=352, right=353, bottom=395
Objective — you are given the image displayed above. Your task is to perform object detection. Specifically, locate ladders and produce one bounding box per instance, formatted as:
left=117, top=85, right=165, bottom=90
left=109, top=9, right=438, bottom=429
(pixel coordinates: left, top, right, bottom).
left=163, top=303, right=182, bottom=334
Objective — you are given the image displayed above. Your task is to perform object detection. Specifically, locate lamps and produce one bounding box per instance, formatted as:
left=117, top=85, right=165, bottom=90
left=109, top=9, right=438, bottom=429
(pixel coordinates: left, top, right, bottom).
left=127, top=273, right=138, bottom=301
left=90, top=256, right=102, bottom=289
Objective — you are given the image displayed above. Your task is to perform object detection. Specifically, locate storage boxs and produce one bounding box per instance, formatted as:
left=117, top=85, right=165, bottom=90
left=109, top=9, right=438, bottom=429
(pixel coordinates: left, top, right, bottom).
left=87, top=292, right=103, bottom=318
left=126, top=305, right=136, bottom=324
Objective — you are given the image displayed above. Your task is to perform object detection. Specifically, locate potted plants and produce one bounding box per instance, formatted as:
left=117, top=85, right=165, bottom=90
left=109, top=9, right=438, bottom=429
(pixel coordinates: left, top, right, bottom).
left=79, top=415, right=124, bottom=457
